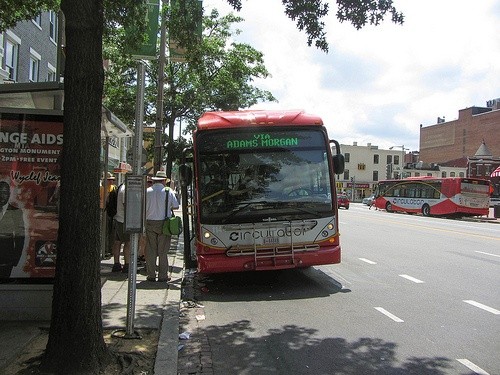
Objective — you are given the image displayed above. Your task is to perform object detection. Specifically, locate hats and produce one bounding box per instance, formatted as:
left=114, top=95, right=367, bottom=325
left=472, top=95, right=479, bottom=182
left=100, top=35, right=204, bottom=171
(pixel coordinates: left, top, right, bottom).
left=102, top=172, right=115, bottom=180
left=152, top=171, right=167, bottom=180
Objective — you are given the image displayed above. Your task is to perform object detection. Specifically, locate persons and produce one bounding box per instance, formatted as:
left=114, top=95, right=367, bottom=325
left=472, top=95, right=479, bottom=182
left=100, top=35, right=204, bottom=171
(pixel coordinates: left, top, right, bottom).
left=100, top=171, right=171, bottom=274
left=0, top=181, right=25, bottom=279
left=139, top=171, right=179, bottom=281
left=368, top=194, right=378, bottom=210
left=207, top=168, right=263, bottom=210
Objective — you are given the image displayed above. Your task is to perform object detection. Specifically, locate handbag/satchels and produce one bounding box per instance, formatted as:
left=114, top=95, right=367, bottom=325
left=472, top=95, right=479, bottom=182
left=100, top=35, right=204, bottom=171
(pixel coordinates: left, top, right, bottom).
left=162, top=216, right=182, bottom=235
left=106, top=184, right=123, bottom=217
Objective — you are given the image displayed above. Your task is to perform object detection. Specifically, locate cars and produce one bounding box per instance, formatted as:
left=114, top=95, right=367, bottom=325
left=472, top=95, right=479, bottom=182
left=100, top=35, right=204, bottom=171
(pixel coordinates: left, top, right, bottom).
left=363, top=194, right=375, bottom=206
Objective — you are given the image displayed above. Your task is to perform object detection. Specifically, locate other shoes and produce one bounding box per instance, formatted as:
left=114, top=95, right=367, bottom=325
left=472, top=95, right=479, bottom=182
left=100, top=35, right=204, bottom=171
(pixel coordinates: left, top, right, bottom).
left=147, top=277, right=156, bottom=281
left=159, top=278, right=171, bottom=282
left=112, top=264, right=122, bottom=272
left=123, top=263, right=140, bottom=273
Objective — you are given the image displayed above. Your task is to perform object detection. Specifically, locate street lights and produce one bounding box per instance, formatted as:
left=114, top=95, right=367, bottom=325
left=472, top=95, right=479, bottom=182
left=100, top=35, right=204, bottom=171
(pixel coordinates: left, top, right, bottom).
left=389, top=145, right=404, bottom=179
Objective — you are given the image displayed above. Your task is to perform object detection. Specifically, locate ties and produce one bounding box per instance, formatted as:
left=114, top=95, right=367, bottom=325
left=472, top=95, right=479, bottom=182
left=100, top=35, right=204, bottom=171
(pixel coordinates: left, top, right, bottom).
left=0, top=207, right=4, bottom=219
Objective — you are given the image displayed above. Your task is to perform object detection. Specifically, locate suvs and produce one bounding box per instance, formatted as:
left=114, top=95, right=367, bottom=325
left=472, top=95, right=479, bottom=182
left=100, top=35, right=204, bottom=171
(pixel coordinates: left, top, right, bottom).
left=337, top=194, right=349, bottom=209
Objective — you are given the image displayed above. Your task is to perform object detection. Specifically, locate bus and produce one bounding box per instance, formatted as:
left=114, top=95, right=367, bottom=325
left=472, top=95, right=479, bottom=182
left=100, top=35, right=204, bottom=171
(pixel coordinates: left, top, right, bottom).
left=177, top=108, right=345, bottom=275
left=374, top=176, right=490, bottom=217
